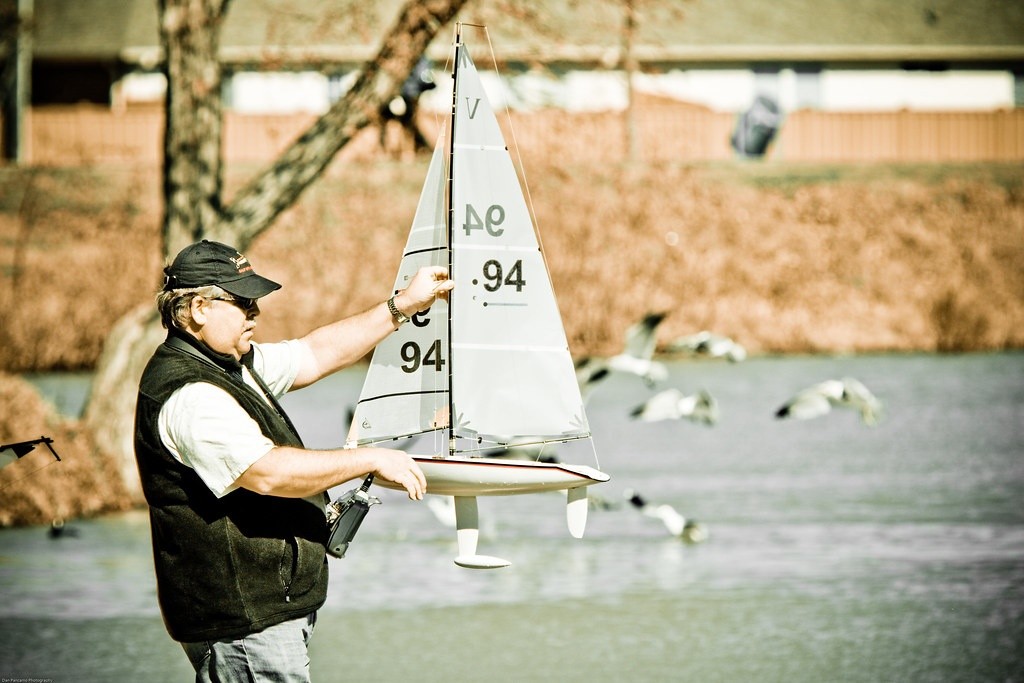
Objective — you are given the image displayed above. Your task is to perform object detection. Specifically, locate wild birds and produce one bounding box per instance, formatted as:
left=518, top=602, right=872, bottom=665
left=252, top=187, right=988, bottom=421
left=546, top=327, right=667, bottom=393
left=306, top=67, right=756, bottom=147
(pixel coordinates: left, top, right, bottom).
left=630, top=387, right=718, bottom=428
left=675, top=329, right=747, bottom=364
left=774, top=378, right=885, bottom=427
left=574, top=308, right=671, bottom=389
left=628, top=494, right=707, bottom=544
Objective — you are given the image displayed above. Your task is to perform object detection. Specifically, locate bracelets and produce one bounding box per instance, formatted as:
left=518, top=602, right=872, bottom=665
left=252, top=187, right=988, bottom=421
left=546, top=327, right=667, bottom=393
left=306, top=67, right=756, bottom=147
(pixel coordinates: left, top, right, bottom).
left=388, top=295, right=411, bottom=323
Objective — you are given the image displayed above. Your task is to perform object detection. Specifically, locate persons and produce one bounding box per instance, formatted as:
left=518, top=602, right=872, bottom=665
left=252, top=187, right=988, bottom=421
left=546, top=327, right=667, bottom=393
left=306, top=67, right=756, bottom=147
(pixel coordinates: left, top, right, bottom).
left=130, top=238, right=455, bottom=682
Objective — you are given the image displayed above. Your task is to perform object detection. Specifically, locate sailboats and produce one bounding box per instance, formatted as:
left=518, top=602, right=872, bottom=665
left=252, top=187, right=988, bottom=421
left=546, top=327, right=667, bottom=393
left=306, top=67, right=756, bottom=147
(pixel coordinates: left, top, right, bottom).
left=339, top=19, right=614, bottom=501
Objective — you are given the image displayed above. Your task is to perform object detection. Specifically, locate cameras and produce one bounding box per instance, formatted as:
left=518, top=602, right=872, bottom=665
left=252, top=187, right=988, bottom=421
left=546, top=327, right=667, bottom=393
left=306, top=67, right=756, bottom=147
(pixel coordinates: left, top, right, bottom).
left=325, top=488, right=381, bottom=558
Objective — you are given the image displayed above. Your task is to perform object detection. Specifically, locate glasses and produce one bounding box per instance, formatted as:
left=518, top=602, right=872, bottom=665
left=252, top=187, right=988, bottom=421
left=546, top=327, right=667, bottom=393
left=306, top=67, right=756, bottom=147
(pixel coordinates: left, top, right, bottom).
left=208, top=297, right=258, bottom=307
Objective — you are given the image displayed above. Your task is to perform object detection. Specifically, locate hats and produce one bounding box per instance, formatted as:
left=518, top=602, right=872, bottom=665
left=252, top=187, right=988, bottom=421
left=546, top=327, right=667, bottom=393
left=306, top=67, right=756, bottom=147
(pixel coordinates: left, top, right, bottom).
left=164, top=239, right=281, bottom=299
